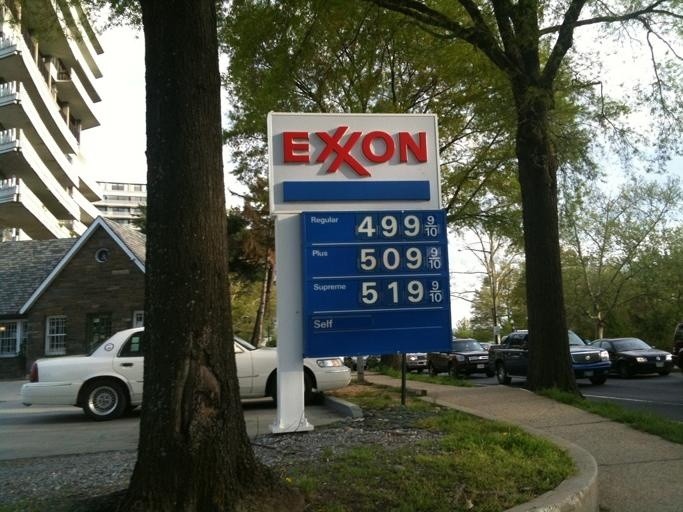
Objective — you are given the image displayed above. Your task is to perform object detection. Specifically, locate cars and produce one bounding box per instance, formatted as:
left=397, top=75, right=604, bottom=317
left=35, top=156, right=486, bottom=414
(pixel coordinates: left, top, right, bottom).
left=20, top=326, right=352, bottom=422
